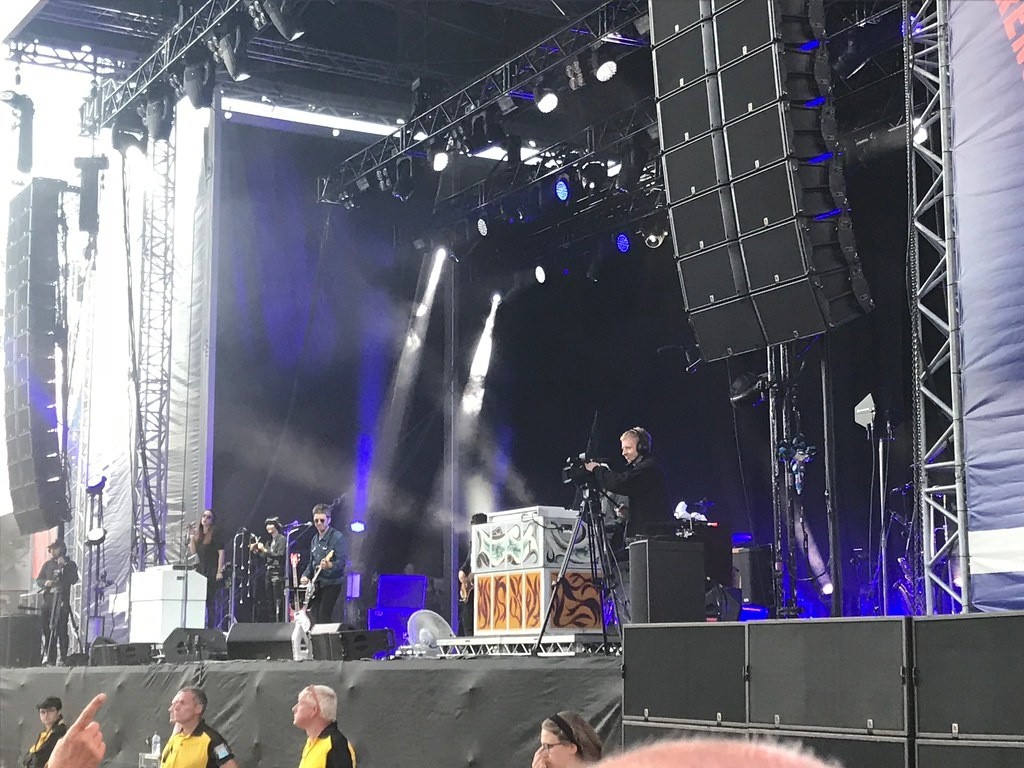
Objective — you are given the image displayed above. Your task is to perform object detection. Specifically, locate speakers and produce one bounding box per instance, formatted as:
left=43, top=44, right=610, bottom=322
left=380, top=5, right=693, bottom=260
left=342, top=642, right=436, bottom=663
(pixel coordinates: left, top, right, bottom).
left=627, top=540, right=708, bottom=624
left=224, top=622, right=312, bottom=661
left=0, top=612, right=42, bottom=669
left=647, top=0, right=875, bottom=362
left=730, top=551, right=773, bottom=607
left=6, top=178, right=69, bottom=533
left=309, top=623, right=351, bottom=660
left=163, top=628, right=228, bottom=663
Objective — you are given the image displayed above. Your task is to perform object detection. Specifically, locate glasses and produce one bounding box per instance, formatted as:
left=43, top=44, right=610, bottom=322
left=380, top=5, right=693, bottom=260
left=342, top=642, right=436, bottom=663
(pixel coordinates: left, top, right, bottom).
left=315, top=518, right=328, bottom=523
left=541, top=742, right=571, bottom=748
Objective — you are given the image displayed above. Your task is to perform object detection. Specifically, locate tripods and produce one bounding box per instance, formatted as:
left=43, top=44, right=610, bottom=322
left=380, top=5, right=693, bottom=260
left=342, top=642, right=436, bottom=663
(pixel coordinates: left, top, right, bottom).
left=531, top=482, right=624, bottom=657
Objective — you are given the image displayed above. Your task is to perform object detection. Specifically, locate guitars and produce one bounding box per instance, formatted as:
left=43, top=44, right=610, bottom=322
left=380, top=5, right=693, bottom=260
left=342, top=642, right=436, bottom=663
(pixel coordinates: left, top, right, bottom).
left=290, top=552, right=311, bottom=633
left=303, top=549, right=335, bottom=612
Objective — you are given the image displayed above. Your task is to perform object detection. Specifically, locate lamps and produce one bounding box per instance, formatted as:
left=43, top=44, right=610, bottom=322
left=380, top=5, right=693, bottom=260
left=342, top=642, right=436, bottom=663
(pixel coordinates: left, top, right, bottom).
left=579, top=160, right=607, bottom=194
left=530, top=256, right=554, bottom=287
left=639, top=214, right=671, bottom=250
left=496, top=199, right=518, bottom=226
left=216, top=33, right=254, bottom=83
left=462, top=113, right=508, bottom=158
left=590, top=51, right=622, bottom=84
left=261, top=0, right=307, bottom=43
left=372, top=167, right=393, bottom=194
left=143, top=87, right=175, bottom=143
left=581, top=239, right=608, bottom=286
left=181, top=52, right=217, bottom=112
left=615, top=230, right=642, bottom=255
left=563, top=56, right=588, bottom=92
left=492, top=95, right=522, bottom=119
left=475, top=211, right=491, bottom=237
left=423, top=146, right=451, bottom=173
left=390, top=159, right=418, bottom=203
left=333, top=187, right=361, bottom=217
left=351, top=174, right=370, bottom=194
left=530, top=82, right=580, bottom=116
left=614, top=137, right=647, bottom=194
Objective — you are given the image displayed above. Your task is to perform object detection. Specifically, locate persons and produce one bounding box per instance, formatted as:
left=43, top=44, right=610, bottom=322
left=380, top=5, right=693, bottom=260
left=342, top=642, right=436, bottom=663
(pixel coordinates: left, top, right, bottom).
left=48, top=694, right=106, bottom=768
left=301, top=504, right=347, bottom=623
left=292, top=685, right=357, bottom=768
left=254, top=517, right=287, bottom=623
left=36, top=541, right=79, bottom=665
left=585, top=427, right=671, bottom=538
left=23, top=697, right=67, bottom=768
left=160, top=688, right=239, bottom=768
left=188, top=509, right=225, bottom=629
left=593, top=740, right=828, bottom=768
left=532, top=711, right=602, bottom=768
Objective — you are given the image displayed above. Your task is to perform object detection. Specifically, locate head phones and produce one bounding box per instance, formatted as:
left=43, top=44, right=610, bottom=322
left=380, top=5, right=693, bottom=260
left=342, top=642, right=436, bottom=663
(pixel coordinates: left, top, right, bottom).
left=630, top=425, right=650, bottom=455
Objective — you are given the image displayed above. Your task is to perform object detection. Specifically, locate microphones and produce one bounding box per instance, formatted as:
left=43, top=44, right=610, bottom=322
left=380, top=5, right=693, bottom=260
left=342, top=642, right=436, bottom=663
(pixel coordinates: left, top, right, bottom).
left=293, top=521, right=312, bottom=529
left=183, top=519, right=196, bottom=529
left=243, top=527, right=256, bottom=537
left=284, top=520, right=298, bottom=528
